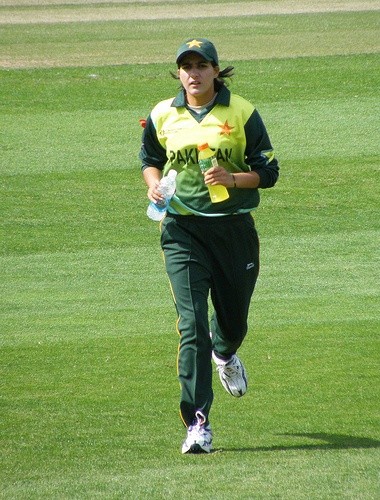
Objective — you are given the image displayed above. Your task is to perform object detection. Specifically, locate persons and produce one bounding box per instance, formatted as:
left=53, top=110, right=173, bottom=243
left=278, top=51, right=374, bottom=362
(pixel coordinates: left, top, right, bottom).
left=138, top=38, right=277, bottom=455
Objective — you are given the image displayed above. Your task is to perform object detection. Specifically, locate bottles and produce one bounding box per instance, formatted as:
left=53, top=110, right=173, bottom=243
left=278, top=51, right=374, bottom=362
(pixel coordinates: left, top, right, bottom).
left=197, top=142, right=230, bottom=204
left=146, top=169, right=178, bottom=220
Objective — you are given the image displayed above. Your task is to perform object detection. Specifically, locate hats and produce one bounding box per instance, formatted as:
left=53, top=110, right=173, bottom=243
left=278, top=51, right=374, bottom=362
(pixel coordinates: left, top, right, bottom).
left=176, top=38, right=218, bottom=67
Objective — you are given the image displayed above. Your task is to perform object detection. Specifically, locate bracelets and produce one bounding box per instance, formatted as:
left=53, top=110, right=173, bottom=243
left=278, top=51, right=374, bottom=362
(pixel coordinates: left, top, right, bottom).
left=231, top=173, right=239, bottom=190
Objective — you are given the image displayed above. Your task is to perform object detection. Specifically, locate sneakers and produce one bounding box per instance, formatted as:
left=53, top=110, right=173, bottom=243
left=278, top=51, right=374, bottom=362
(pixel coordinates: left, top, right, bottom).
left=210, top=349, right=248, bottom=397
left=182, top=425, right=213, bottom=453
